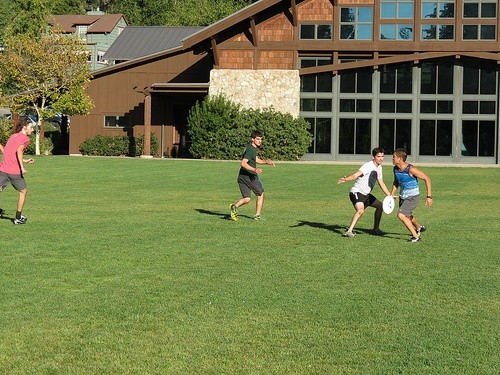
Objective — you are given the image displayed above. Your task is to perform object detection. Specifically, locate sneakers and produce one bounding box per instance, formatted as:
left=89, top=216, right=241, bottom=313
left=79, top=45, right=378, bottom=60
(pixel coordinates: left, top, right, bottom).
left=254, top=215, right=267, bottom=221
left=14, top=217, right=27, bottom=225
left=229, top=203, right=238, bottom=221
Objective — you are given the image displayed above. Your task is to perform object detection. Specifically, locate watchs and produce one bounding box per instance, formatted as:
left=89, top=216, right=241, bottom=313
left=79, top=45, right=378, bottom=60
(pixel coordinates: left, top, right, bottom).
left=427, top=196, right=433, bottom=199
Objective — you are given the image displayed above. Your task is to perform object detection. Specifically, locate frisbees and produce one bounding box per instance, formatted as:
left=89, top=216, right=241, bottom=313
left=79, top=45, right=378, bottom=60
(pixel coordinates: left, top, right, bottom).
left=382, top=195, right=395, bottom=215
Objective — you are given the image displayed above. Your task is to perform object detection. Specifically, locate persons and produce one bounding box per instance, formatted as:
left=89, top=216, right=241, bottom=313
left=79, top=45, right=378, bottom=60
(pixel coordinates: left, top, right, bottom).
left=391, top=149, right=432, bottom=242
left=0, top=117, right=34, bottom=225
left=229, top=131, right=272, bottom=222
left=336, top=147, right=391, bottom=238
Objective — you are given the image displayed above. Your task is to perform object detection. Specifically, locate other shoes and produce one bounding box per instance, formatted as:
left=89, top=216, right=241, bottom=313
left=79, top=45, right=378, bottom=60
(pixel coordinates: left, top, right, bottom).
left=408, top=225, right=426, bottom=242
left=372, top=228, right=386, bottom=235
left=344, top=230, right=356, bottom=238
